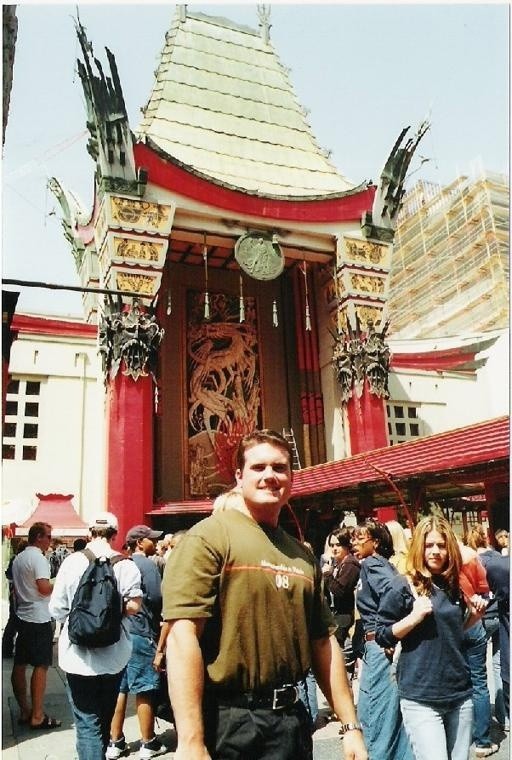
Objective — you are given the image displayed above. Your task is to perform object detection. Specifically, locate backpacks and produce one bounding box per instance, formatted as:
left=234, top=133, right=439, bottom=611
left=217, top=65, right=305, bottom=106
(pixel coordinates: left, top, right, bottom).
left=66, top=548, right=134, bottom=649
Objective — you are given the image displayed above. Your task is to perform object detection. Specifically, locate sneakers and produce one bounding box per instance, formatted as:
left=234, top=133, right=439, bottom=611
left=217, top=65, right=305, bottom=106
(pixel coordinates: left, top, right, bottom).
left=473, top=722, right=510, bottom=759
left=105, top=736, right=131, bottom=760
left=138, top=736, right=169, bottom=760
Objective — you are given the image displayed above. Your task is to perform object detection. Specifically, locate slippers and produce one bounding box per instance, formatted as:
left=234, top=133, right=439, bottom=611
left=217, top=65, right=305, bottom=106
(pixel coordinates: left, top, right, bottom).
left=17, top=709, right=62, bottom=729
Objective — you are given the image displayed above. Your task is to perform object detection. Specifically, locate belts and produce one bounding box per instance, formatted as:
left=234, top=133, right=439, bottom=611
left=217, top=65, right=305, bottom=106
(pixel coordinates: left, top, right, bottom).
left=202, top=683, right=302, bottom=711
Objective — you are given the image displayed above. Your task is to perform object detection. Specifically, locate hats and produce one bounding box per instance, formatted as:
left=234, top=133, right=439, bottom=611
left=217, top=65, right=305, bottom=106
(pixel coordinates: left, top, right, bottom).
left=88, top=512, right=118, bottom=532
left=125, top=525, right=164, bottom=544
